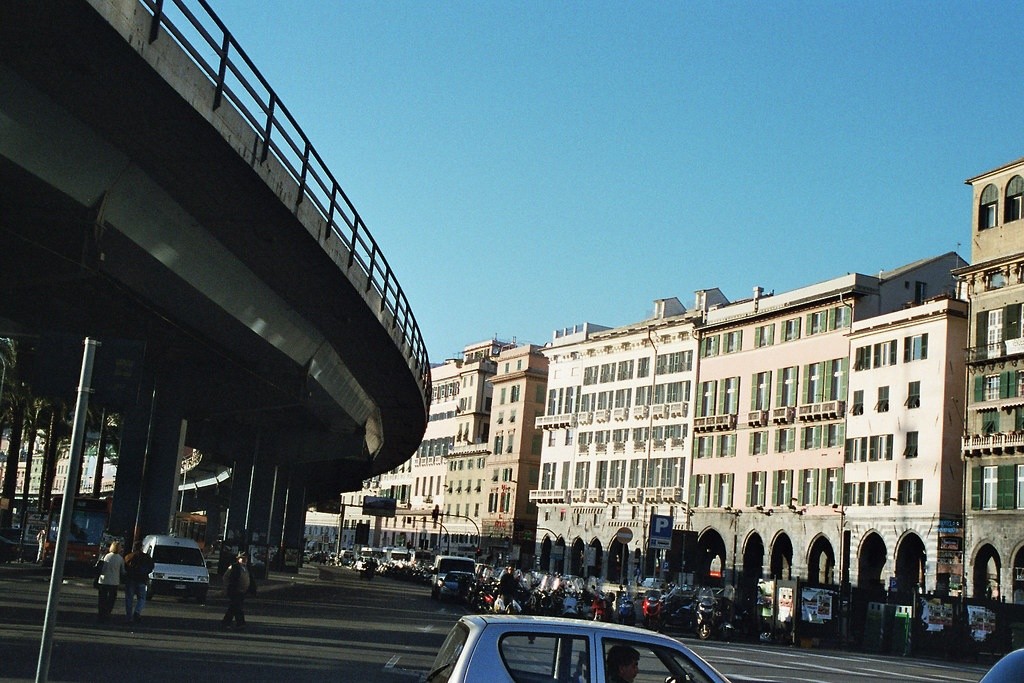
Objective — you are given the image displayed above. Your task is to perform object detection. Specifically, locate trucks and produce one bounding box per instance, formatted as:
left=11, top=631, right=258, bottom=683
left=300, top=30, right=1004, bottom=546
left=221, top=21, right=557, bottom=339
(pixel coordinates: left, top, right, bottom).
left=39, top=495, right=113, bottom=578
left=21, top=511, right=46, bottom=559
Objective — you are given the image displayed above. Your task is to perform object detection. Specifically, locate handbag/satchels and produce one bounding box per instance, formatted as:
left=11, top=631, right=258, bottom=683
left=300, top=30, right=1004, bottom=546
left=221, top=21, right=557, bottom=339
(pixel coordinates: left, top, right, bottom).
left=129, top=554, right=155, bottom=581
left=493, top=596, right=505, bottom=613
left=512, top=599, right=523, bottom=614
left=94, top=553, right=106, bottom=572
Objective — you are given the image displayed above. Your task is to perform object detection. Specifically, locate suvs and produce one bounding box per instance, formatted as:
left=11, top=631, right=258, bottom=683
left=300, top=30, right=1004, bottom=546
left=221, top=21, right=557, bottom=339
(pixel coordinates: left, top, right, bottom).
left=352, top=557, right=367, bottom=572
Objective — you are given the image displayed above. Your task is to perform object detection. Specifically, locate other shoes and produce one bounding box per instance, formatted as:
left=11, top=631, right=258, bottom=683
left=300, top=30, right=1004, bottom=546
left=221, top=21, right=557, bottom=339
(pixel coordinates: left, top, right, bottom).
left=134, top=618, right=145, bottom=623
left=222, top=624, right=231, bottom=630
left=238, top=626, right=249, bottom=630
left=97, top=614, right=111, bottom=618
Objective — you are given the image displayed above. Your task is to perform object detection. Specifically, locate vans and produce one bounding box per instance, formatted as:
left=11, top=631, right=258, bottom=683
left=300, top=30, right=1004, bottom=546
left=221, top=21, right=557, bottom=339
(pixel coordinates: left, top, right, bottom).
left=340, top=550, right=352, bottom=564
left=139, top=534, right=209, bottom=603
left=303, top=550, right=310, bottom=563
left=430, top=555, right=475, bottom=597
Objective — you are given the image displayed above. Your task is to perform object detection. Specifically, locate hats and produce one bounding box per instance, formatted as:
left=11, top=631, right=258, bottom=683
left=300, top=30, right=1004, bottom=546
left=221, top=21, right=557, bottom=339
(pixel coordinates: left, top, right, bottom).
left=236, top=550, right=249, bottom=559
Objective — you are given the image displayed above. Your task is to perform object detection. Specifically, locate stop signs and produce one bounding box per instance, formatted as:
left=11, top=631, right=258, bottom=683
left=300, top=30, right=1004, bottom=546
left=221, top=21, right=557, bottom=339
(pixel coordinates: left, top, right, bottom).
left=616, top=527, right=633, bottom=544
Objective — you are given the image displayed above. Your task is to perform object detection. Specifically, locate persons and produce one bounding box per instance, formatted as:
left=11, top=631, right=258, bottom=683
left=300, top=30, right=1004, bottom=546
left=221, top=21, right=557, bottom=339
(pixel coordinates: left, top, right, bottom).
left=498, top=566, right=519, bottom=615
left=606, top=644, right=641, bottom=683
left=362, top=558, right=378, bottom=578
left=96, top=540, right=155, bottom=622
left=35, top=526, right=47, bottom=564
left=220, top=550, right=252, bottom=629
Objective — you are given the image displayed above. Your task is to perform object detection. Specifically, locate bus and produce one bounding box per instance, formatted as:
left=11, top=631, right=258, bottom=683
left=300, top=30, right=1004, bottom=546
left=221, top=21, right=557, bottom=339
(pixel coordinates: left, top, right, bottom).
left=383, top=547, right=432, bottom=562
left=361, top=548, right=386, bottom=562
left=171, top=512, right=208, bottom=550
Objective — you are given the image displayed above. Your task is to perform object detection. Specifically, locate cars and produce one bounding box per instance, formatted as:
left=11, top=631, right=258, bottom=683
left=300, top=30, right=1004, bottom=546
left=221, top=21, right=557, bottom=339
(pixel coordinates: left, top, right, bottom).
left=439, top=572, right=475, bottom=603
left=423, top=614, right=731, bottom=683
left=0, top=535, right=24, bottom=564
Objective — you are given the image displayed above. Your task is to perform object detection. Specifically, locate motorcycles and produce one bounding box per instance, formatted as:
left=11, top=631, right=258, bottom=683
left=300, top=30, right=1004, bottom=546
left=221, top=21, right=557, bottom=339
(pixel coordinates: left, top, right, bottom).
left=377, top=561, right=752, bottom=641
